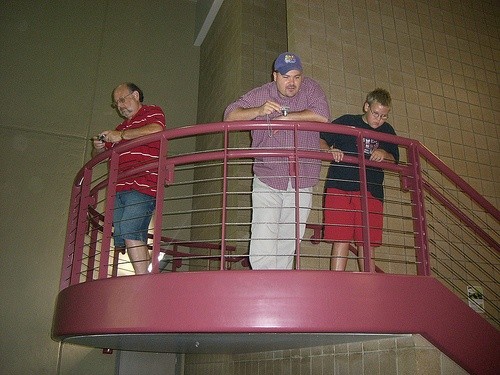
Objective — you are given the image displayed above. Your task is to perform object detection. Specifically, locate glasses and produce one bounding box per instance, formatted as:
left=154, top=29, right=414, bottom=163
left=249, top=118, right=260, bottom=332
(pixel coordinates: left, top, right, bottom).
left=111, top=92, right=133, bottom=109
left=369, top=104, right=391, bottom=120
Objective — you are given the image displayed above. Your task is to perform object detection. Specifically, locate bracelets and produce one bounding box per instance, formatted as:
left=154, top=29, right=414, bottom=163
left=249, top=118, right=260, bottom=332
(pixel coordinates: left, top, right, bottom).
left=120, top=129, right=127, bottom=140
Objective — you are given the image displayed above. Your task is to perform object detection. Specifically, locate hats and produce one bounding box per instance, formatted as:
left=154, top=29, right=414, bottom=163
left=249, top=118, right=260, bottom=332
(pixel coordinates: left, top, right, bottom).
left=273, top=52, right=303, bottom=75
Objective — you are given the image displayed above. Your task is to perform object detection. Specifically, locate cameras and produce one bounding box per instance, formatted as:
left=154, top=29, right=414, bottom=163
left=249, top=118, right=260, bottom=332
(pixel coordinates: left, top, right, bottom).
left=97, top=135, right=106, bottom=143
left=280, top=106, right=290, bottom=116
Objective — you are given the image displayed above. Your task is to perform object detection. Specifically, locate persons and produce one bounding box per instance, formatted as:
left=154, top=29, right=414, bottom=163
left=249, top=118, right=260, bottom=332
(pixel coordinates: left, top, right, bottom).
left=224, top=53, right=330, bottom=270
left=90, top=83, right=165, bottom=275
left=320, top=88, right=399, bottom=273
